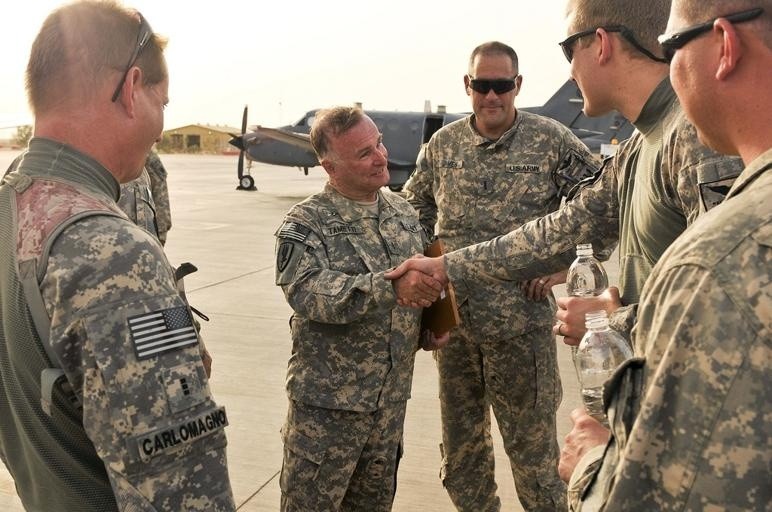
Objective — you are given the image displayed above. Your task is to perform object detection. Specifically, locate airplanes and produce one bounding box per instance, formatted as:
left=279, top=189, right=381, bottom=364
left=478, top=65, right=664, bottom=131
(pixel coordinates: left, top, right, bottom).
left=224, top=72, right=638, bottom=198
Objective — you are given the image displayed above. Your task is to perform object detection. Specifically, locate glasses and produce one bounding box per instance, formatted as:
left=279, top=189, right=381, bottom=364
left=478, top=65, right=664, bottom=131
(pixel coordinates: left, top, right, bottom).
left=558, top=25, right=666, bottom=64
left=658, top=7, right=763, bottom=61
left=111, top=12, right=154, bottom=102
left=466, top=73, right=518, bottom=95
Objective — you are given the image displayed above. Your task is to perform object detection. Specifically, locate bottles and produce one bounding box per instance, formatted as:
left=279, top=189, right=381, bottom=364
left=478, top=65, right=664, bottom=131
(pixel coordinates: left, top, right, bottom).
left=566, top=241, right=610, bottom=374
left=573, top=309, right=630, bottom=433
